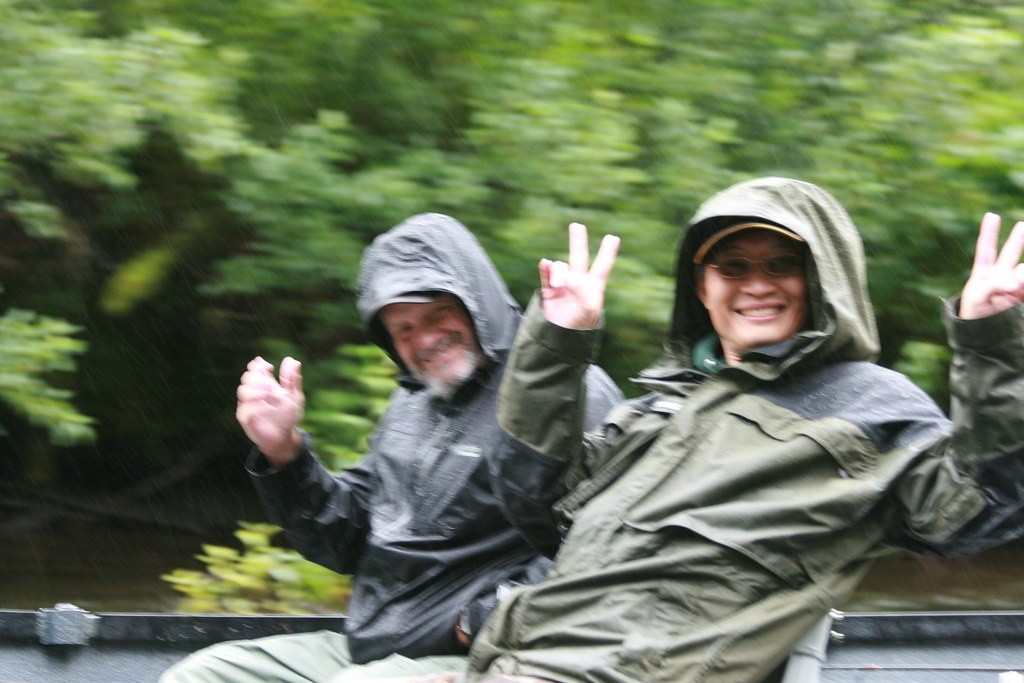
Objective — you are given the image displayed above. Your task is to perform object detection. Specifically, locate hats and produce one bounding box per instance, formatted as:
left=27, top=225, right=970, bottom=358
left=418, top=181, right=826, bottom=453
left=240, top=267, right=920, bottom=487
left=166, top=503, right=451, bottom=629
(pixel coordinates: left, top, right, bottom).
left=691, top=218, right=805, bottom=264
left=362, top=289, right=441, bottom=323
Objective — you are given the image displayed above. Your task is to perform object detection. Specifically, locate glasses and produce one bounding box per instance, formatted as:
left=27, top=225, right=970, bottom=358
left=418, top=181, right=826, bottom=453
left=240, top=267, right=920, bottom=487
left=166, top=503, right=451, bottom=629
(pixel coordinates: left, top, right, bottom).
left=703, top=257, right=804, bottom=278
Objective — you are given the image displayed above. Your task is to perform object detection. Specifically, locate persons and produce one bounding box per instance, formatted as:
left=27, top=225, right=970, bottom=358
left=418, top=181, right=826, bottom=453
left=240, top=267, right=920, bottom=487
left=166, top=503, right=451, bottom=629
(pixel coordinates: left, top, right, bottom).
left=159, top=213, right=621, bottom=683
left=464, top=177, right=1023, bottom=682
